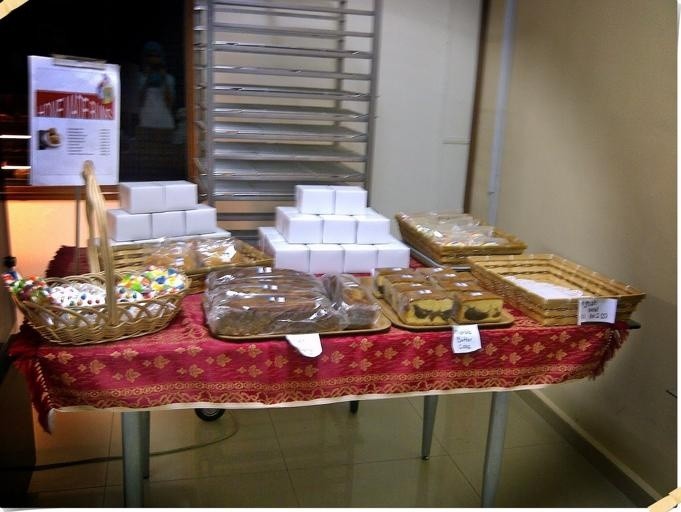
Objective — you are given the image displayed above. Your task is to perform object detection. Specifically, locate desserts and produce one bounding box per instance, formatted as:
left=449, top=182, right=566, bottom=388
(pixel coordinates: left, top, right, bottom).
left=146, top=239, right=502, bottom=336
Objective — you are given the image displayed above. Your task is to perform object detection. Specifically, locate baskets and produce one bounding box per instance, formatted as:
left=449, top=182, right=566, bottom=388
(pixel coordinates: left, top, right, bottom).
left=394, top=210, right=526, bottom=265
left=8, top=159, right=192, bottom=346
left=96, top=232, right=271, bottom=296
left=470, top=251, right=647, bottom=326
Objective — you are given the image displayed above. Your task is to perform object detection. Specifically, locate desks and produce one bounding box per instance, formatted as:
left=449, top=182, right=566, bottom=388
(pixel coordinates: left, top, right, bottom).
left=9, top=242, right=644, bottom=506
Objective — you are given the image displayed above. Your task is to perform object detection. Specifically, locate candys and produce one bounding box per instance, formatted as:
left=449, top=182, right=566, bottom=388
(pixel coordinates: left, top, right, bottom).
left=1, top=266, right=185, bottom=325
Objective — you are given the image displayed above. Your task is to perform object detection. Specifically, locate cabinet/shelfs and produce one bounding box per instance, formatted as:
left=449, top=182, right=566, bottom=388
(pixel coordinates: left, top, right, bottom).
left=190, top=1, right=384, bottom=246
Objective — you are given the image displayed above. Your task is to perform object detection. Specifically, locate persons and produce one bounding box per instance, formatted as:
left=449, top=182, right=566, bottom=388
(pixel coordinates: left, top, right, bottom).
left=128, top=46, right=180, bottom=182
